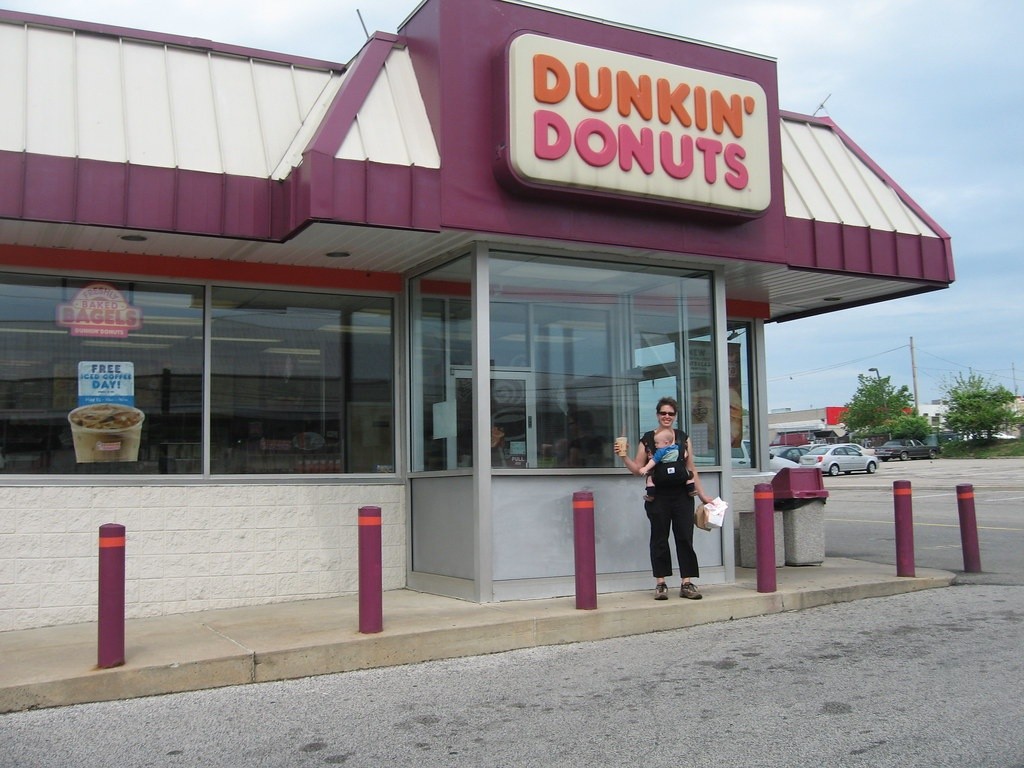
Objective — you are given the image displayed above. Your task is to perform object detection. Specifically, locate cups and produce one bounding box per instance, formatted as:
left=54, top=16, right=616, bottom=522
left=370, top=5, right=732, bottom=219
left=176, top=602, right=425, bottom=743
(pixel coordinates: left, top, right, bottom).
left=616, top=437, right=629, bottom=457
left=68, top=404, right=144, bottom=463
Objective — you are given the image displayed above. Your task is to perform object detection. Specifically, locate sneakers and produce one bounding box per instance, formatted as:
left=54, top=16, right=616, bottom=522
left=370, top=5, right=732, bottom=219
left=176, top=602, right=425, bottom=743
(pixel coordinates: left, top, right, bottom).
left=680, top=582, right=702, bottom=599
left=654, top=582, right=669, bottom=600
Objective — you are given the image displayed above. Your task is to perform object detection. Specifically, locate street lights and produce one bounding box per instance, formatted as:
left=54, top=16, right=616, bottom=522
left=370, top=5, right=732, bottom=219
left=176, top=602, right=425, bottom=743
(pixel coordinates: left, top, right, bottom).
left=868, top=368, right=893, bottom=441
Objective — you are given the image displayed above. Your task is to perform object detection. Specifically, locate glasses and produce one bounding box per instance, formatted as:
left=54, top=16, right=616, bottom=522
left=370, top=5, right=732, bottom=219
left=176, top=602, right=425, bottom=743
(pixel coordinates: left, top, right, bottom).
left=658, top=411, right=676, bottom=416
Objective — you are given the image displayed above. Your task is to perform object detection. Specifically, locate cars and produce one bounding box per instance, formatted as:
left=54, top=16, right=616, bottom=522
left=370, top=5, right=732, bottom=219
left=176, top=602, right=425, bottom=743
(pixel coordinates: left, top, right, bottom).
left=964, top=430, right=1019, bottom=441
left=874, top=439, right=942, bottom=461
left=694, top=438, right=800, bottom=474
left=770, top=446, right=812, bottom=463
left=798, top=443, right=828, bottom=451
left=939, top=431, right=964, bottom=443
left=834, top=443, right=877, bottom=457
left=799, top=446, right=880, bottom=475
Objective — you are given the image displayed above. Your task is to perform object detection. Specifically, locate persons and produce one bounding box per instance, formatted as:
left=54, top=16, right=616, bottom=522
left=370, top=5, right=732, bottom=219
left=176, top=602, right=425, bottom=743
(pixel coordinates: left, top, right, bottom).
left=614, top=398, right=715, bottom=599
left=549, top=411, right=609, bottom=466
left=638, top=427, right=698, bottom=502
